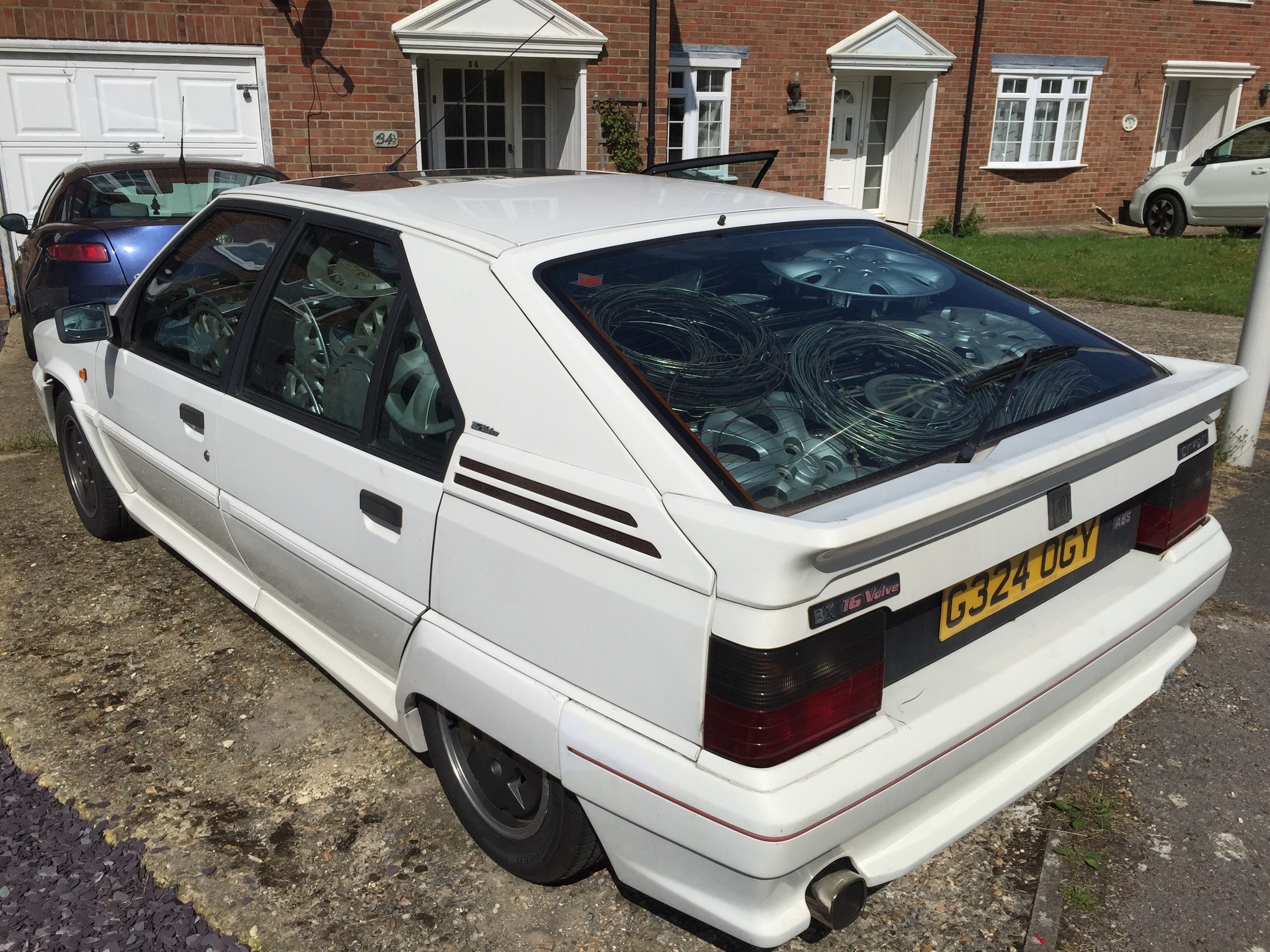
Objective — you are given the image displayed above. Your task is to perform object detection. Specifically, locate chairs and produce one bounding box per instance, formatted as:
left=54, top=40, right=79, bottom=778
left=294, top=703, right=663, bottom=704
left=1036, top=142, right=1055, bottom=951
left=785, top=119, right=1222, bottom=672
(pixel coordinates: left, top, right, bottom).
left=110, top=203, right=149, bottom=216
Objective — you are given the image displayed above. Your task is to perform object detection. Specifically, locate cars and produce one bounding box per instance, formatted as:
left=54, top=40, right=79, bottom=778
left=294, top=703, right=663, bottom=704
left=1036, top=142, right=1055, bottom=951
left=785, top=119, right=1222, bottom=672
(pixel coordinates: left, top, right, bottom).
left=1129, top=117, right=1270, bottom=239
left=0, top=155, right=291, bottom=361
left=31, top=151, right=1251, bottom=945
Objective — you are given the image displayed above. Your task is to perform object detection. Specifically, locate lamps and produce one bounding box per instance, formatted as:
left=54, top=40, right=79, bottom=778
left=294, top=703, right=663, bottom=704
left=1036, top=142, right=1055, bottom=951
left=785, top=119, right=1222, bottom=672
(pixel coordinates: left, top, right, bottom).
left=786, top=68, right=805, bottom=102
